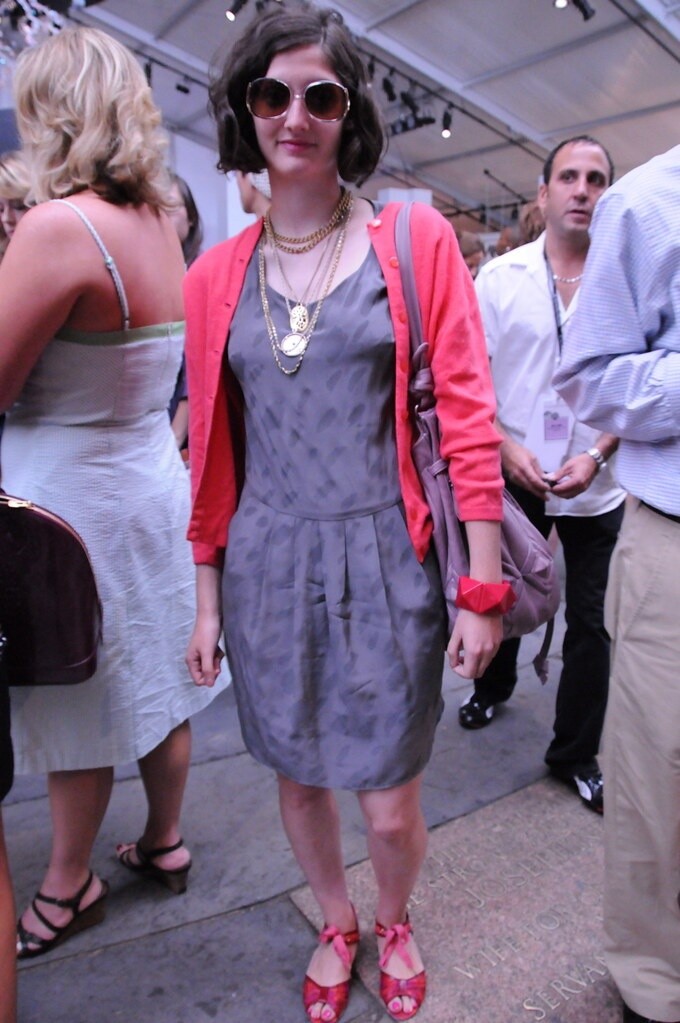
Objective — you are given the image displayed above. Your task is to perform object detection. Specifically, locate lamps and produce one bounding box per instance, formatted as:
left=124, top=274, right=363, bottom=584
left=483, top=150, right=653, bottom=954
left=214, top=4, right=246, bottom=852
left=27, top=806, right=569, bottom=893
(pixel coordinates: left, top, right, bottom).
left=570, top=0, right=598, bottom=21
left=221, top=2, right=454, bottom=138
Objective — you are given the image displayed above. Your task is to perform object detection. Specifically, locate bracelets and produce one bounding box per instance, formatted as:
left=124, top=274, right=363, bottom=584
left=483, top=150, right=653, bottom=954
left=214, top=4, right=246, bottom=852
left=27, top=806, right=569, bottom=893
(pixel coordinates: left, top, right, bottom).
left=584, top=448, right=604, bottom=466
left=455, top=576, right=518, bottom=618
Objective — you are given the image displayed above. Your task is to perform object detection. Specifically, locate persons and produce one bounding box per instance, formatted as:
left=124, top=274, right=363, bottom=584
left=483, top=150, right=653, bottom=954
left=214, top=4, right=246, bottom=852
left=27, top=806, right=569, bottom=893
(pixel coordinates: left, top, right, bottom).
left=186, top=4, right=506, bottom=1023
left=456, top=231, right=486, bottom=281
left=0, top=24, right=233, bottom=1023
left=497, top=202, right=545, bottom=257
left=551, top=142, right=680, bottom=1023
left=456, top=134, right=625, bottom=811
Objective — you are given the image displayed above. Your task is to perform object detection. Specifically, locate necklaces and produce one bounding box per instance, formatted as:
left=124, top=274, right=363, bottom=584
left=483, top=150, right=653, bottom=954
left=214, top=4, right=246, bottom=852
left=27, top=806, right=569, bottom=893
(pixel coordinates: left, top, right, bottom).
left=257, top=187, right=355, bottom=376
left=551, top=273, right=584, bottom=282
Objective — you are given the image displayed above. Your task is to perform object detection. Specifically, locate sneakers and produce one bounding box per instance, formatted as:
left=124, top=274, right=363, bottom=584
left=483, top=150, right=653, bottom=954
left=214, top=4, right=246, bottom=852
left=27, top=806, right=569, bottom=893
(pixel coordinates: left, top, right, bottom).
left=459, top=691, right=496, bottom=730
left=549, top=758, right=604, bottom=815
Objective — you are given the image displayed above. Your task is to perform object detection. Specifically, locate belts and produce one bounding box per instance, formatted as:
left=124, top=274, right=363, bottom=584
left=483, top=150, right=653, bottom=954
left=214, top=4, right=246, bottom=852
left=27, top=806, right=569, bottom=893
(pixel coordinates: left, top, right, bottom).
left=640, top=500, right=680, bottom=524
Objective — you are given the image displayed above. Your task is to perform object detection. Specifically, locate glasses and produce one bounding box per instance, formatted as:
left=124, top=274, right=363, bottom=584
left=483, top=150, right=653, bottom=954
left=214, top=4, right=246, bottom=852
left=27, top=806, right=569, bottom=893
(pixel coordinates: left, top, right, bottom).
left=241, top=77, right=351, bottom=121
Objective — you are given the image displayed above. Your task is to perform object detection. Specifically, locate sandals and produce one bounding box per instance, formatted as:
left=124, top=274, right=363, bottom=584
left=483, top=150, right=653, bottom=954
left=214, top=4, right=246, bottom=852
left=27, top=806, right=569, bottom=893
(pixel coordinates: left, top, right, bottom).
left=303, top=900, right=360, bottom=1023
left=374, top=910, right=427, bottom=1021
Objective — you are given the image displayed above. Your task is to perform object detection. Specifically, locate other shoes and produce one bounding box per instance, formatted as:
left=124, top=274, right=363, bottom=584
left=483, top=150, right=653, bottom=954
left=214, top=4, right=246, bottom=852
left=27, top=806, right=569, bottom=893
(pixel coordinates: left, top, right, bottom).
left=622, top=999, right=680, bottom=1023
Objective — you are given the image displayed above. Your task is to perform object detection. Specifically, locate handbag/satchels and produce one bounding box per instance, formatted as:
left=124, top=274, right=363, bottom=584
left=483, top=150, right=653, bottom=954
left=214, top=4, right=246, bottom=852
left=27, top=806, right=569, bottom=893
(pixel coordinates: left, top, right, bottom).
left=0, top=489, right=103, bottom=687
left=394, top=201, right=560, bottom=643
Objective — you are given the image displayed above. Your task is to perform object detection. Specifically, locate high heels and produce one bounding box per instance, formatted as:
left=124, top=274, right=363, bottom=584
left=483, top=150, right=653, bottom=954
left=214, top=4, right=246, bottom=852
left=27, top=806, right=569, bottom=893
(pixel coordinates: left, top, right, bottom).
left=119, top=834, right=192, bottom=894
left=15, top=870, right=109, bottom=959
